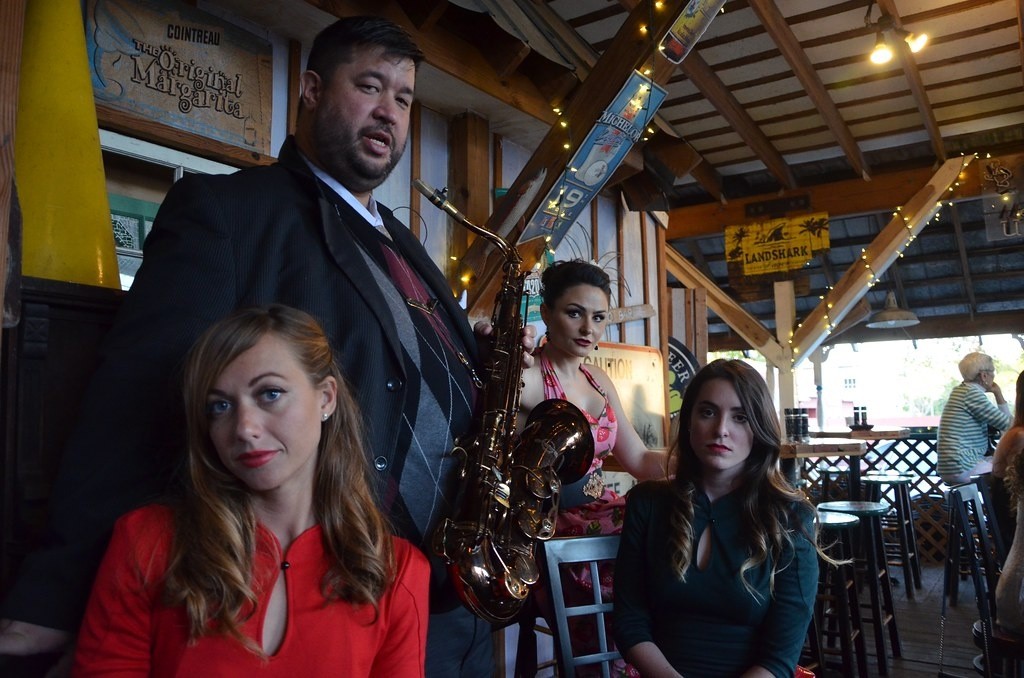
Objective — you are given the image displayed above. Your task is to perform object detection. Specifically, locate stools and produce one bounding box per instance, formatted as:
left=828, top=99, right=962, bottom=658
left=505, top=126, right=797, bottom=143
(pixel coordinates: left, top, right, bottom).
left=943, top=480, right=980, bottom=607
left=815, top=500, right=902, bottom=678
left=816, top=466, right=922, bottom=597
left=798, top=510, right=870, bottom=678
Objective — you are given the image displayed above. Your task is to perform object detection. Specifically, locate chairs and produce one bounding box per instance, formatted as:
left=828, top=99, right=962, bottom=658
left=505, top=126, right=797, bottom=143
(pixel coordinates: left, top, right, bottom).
left=541, top=535, right=623, bottom=678
left=953, top=476, right=1024, bottom=678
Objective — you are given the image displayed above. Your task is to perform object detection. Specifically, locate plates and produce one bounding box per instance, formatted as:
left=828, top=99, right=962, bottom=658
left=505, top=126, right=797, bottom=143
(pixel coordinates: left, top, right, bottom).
left=849, top=425, right=874, bottom=430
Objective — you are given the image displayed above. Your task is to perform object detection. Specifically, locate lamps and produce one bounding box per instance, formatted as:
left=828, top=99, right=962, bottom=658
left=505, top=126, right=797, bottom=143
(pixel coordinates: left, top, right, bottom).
left=865, top=290, right=920, bottom=329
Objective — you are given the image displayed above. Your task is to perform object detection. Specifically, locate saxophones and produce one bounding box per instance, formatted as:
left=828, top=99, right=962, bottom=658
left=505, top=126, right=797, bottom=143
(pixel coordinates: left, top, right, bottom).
left=412, top=176, right=595, bottom=627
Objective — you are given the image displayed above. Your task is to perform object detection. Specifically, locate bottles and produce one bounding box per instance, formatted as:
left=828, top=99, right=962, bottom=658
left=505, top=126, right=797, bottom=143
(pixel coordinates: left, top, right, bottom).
left=854, top=407, right=860, bottom=426
left=802, top=407, right=810, bottom=442
left=784, top=407, right=793, bottom=441
left=792, top=408, right=802, bottom=443
left=861, top=407, right=867, bottom=425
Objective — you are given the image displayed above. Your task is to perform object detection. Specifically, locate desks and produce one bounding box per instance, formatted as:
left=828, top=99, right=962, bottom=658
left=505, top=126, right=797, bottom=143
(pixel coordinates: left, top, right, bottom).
left=778, top=438, right=867, bottom=489
left=808, top=425, right=912, bottom=594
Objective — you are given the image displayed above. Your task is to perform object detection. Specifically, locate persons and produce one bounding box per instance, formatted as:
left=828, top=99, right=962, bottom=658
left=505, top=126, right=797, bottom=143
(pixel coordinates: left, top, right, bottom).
left=0, top=14, right=536, bottom=678
left=992, top=369, right=1024, bottom=630
left=513, top=258, right=682, bottom=678
left=607, top=358, right=854, bottom=678
left=70, top=302, right=430, bottom=678
left=936, top=353, right=1013, bottom=486
